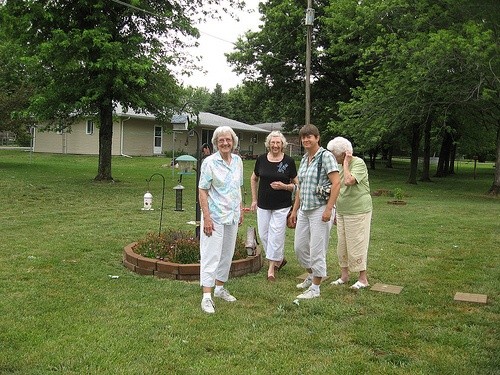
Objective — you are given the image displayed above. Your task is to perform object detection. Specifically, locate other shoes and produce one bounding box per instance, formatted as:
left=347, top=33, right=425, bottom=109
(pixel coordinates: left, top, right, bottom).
left=268, top=271, right=275, bottom=279
left=331, top=278, right=350, bottom=285
left=349, top=280, right=369, bottom=289
left=274, top=259, right=287, bottom=272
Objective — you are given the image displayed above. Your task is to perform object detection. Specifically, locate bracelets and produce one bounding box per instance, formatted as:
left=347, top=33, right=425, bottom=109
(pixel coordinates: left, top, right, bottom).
left=287, top=183, right=288, bottom=190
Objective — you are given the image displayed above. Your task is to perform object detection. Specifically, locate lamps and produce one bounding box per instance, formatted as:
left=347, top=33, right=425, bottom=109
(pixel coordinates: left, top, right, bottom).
left=173, top=184, right=185, bottom=212
left=175, top=147, right=196, bottom=174
left=141, top=190, right=155, bottom=211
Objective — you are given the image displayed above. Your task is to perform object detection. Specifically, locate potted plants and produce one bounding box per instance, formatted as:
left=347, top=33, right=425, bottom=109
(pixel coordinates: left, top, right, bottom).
left=387, top=187, right=407, bottom=205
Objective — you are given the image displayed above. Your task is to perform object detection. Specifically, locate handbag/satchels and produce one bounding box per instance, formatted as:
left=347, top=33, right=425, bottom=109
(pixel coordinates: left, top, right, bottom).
left=287, top=184, right=298, bottom=228
left=316, top=184, right=332, bottom=201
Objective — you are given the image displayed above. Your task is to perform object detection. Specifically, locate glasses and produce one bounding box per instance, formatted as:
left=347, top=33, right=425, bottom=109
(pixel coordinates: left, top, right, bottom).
left=217, top=138, right=233, bottom=143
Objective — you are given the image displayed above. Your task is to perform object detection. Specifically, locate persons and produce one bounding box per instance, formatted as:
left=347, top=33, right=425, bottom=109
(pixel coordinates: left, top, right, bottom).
left=250, top=130, right=299, bottom=282
left=288, top=124, right=341, bottom=298
left=327, top=136, right=374, bottom=291
left=198, top=126, right=243, bottom=313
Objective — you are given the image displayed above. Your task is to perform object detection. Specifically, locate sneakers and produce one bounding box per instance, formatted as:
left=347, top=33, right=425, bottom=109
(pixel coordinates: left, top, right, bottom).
left=296, top=278, right=312, bottom=289
left=200, top=298, right=216, bottom=313
left=213, top=288, right=236, bottom=302
left=296, top=287, right=320, bottom=298
